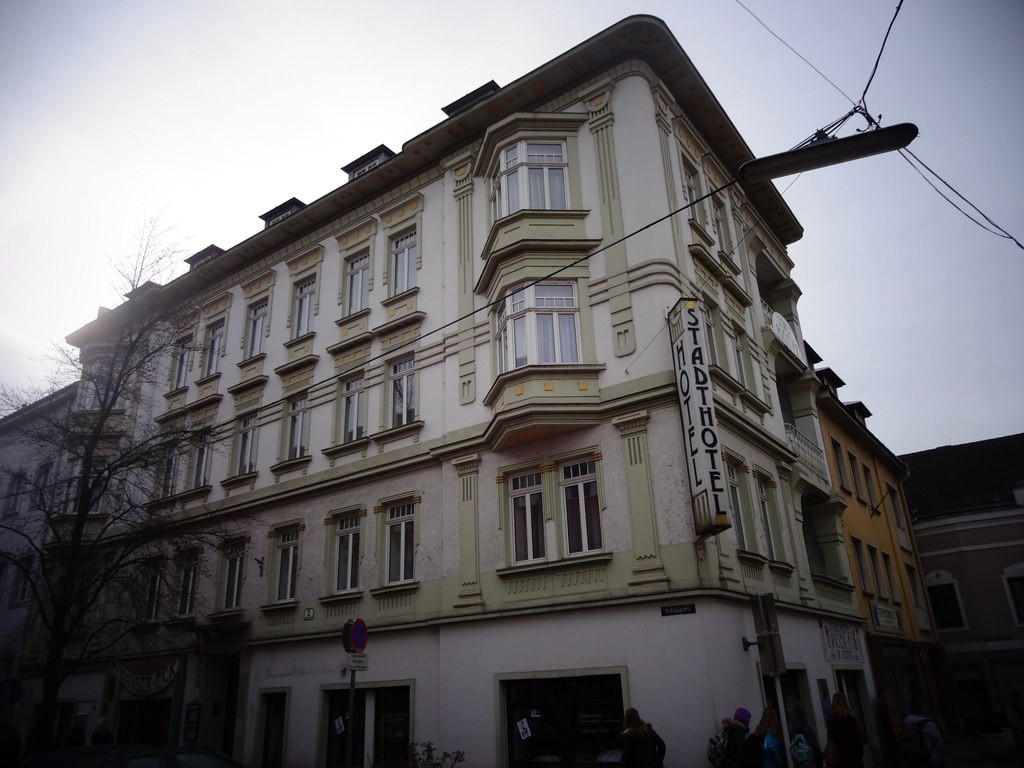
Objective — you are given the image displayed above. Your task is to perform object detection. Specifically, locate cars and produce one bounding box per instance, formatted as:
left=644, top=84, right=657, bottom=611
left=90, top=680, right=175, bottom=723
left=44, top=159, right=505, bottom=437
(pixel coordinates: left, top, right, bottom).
left=15, top=741, right=251, bottom=768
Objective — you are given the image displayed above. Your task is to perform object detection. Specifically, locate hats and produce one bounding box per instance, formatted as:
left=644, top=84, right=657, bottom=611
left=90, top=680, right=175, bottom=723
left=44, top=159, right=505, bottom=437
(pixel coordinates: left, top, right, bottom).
left=733, top=708, right=751, bottom=725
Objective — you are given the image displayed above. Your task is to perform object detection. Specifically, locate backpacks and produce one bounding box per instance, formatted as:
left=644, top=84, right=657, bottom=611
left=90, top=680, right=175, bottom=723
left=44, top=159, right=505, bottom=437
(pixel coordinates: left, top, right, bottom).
left=789, top=734, right=817, bottom=768
left=707, top=731, right=729, bottom=768
left=900, top=719, right=933, bottom=766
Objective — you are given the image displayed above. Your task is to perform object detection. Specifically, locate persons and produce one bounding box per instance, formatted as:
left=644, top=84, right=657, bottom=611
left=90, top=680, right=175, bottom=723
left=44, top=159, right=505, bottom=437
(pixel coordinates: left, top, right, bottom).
left=0, top=717, right=114, bottom=768
left=621, top=707, right=667, bottom=768
left=707, top=693, right=867, bottom=768
left=899, top=700, right=948, bottom=768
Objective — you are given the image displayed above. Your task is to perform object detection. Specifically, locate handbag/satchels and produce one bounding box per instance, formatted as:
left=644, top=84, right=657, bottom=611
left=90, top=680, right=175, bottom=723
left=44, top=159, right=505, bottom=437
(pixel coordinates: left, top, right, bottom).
left=823, top=715, right=839, bottom=768
